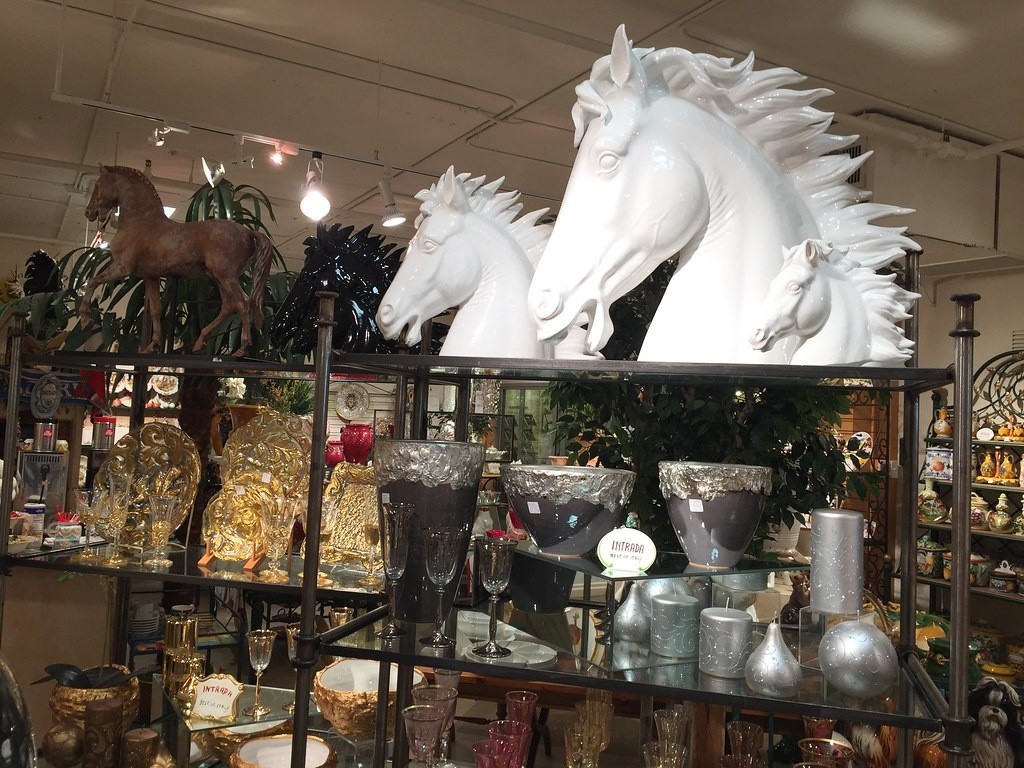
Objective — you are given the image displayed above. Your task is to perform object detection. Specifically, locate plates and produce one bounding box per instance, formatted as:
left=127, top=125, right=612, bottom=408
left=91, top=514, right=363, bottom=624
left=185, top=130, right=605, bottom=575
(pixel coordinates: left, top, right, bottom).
left=93, top=423, right=202, bottom=547
left=218, top=413, right=325, bottom=499
left=323, top=463, right=389, bottom=567
left=105, top=367, right=188, bottom=410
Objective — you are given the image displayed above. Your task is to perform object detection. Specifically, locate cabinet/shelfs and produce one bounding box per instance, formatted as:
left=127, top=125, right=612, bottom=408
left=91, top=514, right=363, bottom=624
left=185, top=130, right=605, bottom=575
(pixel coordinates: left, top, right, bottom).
left=0, top=291, right=1024, bottom=768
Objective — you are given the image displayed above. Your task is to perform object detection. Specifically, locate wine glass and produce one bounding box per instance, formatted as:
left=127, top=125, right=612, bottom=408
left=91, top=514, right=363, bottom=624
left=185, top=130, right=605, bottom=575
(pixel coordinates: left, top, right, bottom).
left=75, top=473, right=864, bottom=767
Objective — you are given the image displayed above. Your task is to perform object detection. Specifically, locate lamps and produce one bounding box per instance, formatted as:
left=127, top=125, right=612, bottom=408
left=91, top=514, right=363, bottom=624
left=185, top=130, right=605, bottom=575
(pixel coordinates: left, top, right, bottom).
left=201, top=133, right=254, bottom=190
left=299, top=152, right=331, bottom=221
left=268, top=142, right=298, bottom=167
left=148, top=119, right=196, bottom=147
left=379, top=165, right=406, bottom=228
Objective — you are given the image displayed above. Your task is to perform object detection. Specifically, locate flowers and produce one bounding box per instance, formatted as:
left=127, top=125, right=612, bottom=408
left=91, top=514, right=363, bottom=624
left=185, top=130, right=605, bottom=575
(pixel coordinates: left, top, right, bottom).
left=213, top=377, right=246, bottom=413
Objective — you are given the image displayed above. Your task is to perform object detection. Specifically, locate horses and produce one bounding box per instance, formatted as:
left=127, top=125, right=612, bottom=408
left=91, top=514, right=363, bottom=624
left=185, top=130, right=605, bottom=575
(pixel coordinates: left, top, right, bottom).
left=525, top=21, right=923, bottom=371
left=268, top=218, right=452, bottom=356
left=77, top=160, right=273, bottom=360
left=375, top=164, right=607, bottom=361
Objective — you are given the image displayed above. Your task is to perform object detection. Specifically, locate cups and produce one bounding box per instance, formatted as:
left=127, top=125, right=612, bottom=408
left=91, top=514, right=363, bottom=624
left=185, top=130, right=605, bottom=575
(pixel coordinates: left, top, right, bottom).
left=169, top=604, right=195, bottom=617
left=372, top=439, right=487, bottom=625
left=35, top=418, right=59, bottom=451
left=93, top=417, right=116, bottom=451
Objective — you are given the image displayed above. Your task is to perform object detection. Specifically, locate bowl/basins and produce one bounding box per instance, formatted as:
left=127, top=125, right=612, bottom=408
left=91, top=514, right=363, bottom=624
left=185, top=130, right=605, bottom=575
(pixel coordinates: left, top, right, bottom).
left=498, top=463, right=637, bottom=558
left=9, top=535, right=39, bottom=554
left=10, top=511, right=31, bottom=529
left=659, top=458, right=771, bottom=571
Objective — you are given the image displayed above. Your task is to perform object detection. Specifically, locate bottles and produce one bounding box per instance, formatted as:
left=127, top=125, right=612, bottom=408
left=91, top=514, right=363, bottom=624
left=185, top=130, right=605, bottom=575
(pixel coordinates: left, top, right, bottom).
left=124, top=727, right=160, bottom=767
left=82, top=698, right=123, bottom=768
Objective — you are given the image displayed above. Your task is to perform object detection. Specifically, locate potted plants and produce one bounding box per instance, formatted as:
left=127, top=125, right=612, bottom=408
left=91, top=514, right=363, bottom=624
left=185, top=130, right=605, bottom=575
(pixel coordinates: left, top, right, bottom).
left=227, top=373, right=315, bottom=435
left=27, top=569, right=163, bottom=768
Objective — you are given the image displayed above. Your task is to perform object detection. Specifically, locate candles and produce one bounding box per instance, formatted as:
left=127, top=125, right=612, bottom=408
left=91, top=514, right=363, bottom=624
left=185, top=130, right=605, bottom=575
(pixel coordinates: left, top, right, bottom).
left=699, top=597, right=753, bottom=678
left=818, top=610, right=898, bottom=699
left=123, top=724, right=159, bottom=768
left=652, top=582, right=699, bottom=658
left=811, top=496, right=864, bottom=614
left=613, top=581, right=654, bottom=644
left=745, top=610, right=802, bottom=698
left=82, top=694, right=123, bottom=768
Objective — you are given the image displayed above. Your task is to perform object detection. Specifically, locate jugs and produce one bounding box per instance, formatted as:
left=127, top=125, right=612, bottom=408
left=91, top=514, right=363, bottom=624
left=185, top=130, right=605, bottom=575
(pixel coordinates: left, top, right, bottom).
left=916, top=445, right=1024, bottom=689
